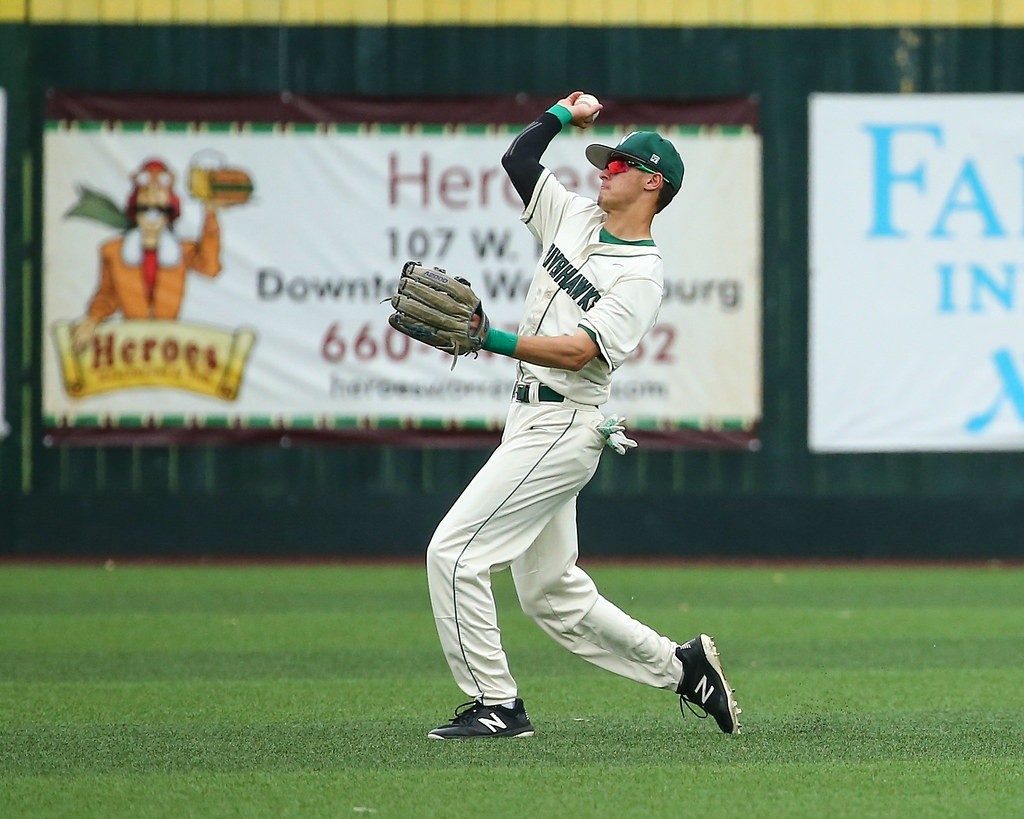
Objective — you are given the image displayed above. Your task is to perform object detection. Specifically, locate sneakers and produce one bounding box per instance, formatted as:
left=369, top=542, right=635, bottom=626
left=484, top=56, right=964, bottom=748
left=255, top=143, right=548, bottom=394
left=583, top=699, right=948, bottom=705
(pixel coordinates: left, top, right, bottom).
left=675, top=633, right=742, bottom=735
left=428, top=697, right=535, bottom=741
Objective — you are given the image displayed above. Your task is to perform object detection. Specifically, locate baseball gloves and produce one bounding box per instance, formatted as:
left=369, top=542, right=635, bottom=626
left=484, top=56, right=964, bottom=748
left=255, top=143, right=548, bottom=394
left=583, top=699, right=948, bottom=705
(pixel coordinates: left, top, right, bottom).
left=388, top=260, right=490, bottom=356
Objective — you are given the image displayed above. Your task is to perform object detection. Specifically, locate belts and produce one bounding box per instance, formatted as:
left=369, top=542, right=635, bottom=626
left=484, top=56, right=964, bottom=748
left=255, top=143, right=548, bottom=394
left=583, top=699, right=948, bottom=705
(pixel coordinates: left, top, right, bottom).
left=514, top=385, right=598, bottom=409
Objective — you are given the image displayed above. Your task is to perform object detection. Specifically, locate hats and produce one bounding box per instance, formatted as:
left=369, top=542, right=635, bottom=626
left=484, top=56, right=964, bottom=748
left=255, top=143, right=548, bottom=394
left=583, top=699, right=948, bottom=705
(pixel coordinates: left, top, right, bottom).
left=585, top=130, right=684, bottom=196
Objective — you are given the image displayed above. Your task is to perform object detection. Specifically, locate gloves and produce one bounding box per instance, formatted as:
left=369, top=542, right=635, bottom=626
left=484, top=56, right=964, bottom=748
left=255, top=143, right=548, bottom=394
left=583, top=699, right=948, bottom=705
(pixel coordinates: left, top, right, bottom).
left=598, top=415, right=638, bottom=455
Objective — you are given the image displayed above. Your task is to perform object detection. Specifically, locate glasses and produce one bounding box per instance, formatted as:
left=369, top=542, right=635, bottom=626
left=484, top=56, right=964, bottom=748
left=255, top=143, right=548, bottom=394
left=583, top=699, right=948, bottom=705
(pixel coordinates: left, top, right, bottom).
left=605, top=156, right=669, bottom=182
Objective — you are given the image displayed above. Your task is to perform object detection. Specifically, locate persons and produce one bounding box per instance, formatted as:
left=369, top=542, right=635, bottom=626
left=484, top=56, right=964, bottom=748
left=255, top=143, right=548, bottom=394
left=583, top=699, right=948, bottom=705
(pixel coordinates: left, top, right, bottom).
left=389, top=90, right=742, bottom=738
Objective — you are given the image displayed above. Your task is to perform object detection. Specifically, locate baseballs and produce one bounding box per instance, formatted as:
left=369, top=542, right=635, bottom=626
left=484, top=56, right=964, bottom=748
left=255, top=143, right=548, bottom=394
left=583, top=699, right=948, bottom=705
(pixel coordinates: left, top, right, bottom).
left=573, top=94, right=600, bottom=122
